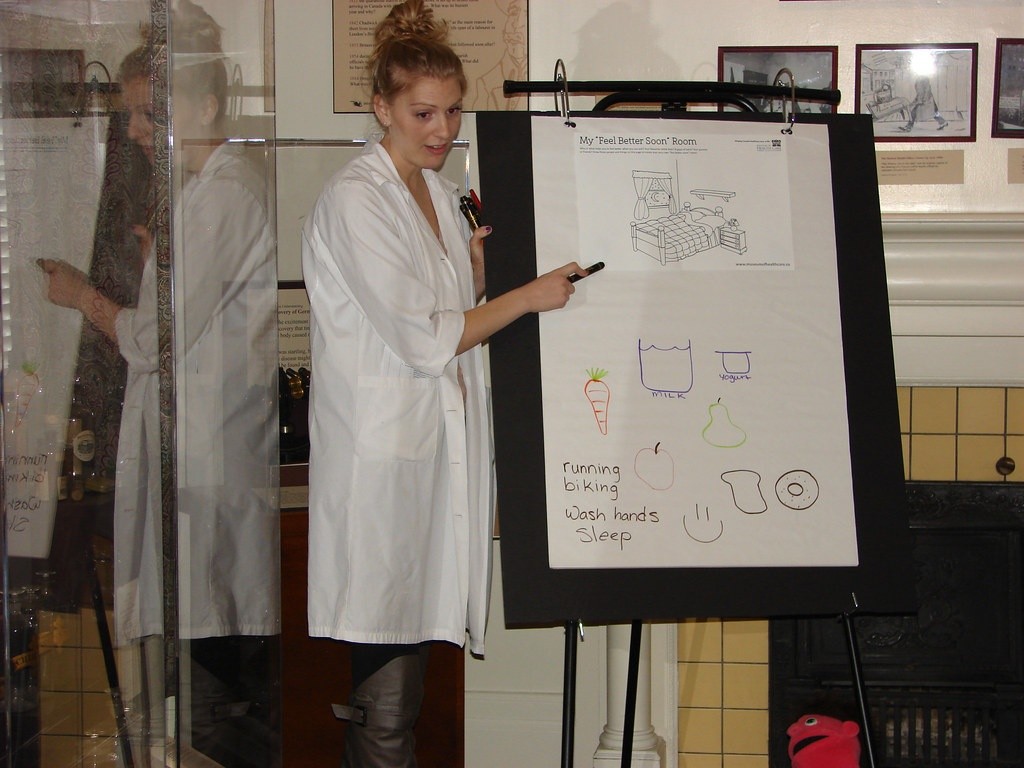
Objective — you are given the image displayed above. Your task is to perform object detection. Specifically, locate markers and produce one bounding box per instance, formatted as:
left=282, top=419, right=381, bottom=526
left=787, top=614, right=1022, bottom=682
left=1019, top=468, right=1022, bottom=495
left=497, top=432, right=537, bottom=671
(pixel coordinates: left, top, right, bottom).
left=458, top=189, right=481, bottom=231
left=36, top=258, right=52, bottom=274
left=568, top=261, right=606, bottom=286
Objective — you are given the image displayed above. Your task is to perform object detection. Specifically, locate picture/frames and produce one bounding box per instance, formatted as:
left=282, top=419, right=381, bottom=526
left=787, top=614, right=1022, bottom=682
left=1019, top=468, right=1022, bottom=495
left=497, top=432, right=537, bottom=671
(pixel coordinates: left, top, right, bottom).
left=717, top=45, right=838, bottom=114
left=991, top=37, right=1024, bottom=139
left=854, top=43, right=977, bottom=143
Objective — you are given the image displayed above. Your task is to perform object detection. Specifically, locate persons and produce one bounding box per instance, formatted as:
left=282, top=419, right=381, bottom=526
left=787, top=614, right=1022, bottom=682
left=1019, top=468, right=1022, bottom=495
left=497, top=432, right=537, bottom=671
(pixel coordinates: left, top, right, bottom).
left=37, top=3, right=283, bottom=768
left=300, top=1, right=587, bottom=767
